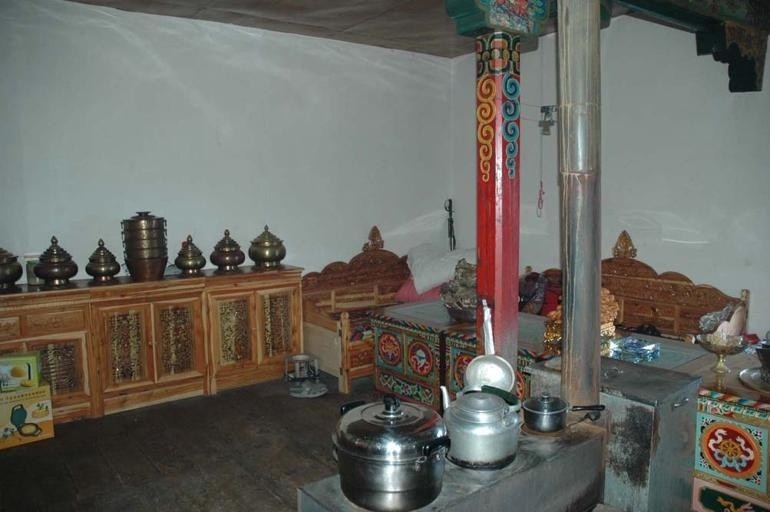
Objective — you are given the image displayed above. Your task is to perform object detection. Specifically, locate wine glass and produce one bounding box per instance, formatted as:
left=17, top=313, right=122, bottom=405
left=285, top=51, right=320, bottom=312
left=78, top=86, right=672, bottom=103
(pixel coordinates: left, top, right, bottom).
left=696, top=333, right=748, bottom=375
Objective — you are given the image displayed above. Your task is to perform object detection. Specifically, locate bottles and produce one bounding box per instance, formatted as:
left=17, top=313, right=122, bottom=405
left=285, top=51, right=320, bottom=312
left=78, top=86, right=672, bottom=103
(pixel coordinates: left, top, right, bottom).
left=24, top=253, right=45, bottom=287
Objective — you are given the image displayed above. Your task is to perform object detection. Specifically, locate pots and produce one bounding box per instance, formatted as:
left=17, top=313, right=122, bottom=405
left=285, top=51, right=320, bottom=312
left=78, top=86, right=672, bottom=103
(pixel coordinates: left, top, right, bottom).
left=331, top=393, right=450, bottom=512
left=523, top=392, right=606, bottom=433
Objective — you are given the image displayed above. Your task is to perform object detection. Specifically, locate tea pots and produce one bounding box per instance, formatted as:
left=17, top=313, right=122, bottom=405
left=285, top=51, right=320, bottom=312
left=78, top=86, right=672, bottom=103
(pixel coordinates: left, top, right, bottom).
left=438, top=385, right=523, bottom=472
